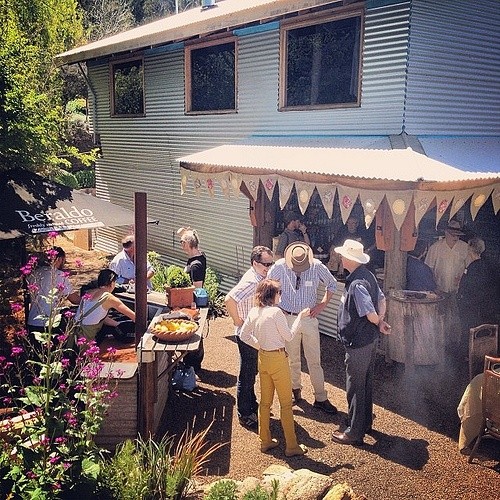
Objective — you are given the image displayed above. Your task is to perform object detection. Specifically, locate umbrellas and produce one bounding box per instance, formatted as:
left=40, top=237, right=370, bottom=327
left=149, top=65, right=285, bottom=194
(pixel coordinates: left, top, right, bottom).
left=0, top=154, right=160, bottom=331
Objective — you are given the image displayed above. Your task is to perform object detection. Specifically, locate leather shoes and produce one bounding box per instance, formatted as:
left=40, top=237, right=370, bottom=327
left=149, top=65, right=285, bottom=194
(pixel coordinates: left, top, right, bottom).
left=332, top=432, right=363, bottom=446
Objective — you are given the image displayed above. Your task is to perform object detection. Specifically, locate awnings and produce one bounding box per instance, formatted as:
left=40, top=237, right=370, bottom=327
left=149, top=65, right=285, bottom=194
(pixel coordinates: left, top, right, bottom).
left=174, top=135, right=500, bottom=182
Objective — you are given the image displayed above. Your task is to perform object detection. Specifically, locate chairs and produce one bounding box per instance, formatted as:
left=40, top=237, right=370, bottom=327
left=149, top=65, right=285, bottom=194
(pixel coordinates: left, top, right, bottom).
left=468, top=355, right=500, bottom=464
left=469, top=324, right=499, bottom=384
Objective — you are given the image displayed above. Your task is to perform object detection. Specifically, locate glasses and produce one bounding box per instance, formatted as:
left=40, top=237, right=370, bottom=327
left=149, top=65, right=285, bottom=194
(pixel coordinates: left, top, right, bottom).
left=276, top=290, right=282, bottom=295
left=256, top=260, right=275, bottom=267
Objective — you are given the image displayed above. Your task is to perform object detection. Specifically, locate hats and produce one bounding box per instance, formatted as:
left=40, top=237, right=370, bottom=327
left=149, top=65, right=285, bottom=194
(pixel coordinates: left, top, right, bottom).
left=284, top=240, right=313, bottom=273
left=334, top=239, right=370, bottom=264
left=446, top=220, right=466, bottom=236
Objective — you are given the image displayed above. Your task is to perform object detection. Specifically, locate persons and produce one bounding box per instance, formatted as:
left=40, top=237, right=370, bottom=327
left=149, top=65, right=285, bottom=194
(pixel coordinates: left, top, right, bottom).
left=266, top=240, right=337, bottom=413
left=406, top=240, right=439, bottom=294
left=28, top=246, right=81, bottom=376
left=453, top=237, right=489, bottom=362
left=52, top=269, right=137, bottom=406
left=238, top=278, right=310, bottom=457
left=332, top=237, right=391, bottom=446
left=425, top=219, right=474, bottom=357
left=274, top=214, right=311, bottom=260
left=177, top=226, right=206, bottom=377
left=108, top=236, right=154, bottom=291
left=225, top=245, right=274, bottom=428
left=74, top=279, right=98, bottom=321
left=330, top=217, right=377, bottom=278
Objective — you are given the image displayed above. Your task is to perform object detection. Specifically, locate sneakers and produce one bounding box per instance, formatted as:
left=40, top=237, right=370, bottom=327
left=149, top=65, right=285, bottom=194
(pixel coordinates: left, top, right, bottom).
left=284, top=444, right=307, bottom=458
left=239, top=406, right=258, bottom=428
left=260, top=439, right=279, bottom=452
left=314, top=399, right=337, bottom=413
left=292, top=397, right=302, bottom=407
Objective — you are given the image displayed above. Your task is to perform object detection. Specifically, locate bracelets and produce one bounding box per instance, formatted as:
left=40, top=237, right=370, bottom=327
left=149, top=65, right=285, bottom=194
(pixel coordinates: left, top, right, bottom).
left=367, top=247, right=371, bottom=253
left=303, top=232, right=307, bottom=234
